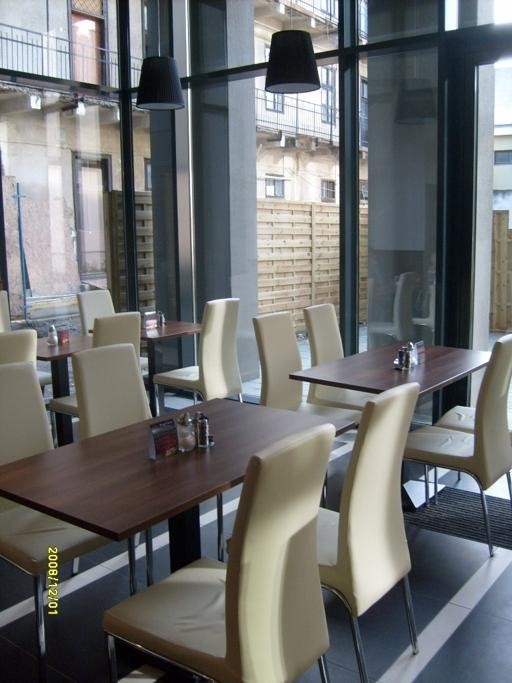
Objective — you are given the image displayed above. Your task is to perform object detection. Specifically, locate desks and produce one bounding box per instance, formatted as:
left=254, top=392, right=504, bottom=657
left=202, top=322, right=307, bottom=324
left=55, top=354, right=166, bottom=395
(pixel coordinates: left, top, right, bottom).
left=37, top=316, right=203, bottom=447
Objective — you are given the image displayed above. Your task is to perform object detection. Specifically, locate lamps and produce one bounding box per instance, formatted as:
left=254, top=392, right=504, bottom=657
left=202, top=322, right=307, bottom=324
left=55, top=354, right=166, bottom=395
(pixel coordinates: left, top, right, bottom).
left=134, top=0, right=187, bottom=112
left=263, top=0, right=322, bottom=94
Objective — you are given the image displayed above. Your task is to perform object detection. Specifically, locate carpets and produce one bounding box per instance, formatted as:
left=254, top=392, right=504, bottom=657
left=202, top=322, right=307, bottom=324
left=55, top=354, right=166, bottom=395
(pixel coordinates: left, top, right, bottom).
left=400, top=484, right=512, bottom=550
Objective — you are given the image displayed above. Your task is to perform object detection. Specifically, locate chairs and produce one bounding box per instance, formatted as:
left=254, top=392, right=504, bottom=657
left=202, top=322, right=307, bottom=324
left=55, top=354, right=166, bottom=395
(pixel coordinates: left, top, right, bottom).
left=432, top=403, right=476, bottom=482
left=250, top=310, right=365, bottom=511
left=76, top=289, right=118, bottom=336
left=0, top=358, right=142, bottom=660
left=100, top=420, right=337, bottom=683
left=1, top=329, right=38, bottom=375
left=44, top=310, right=142, bottom=448
left=366, top=268, right=419, bottom=345
left=400, top=332, right=512, bottom=558
left=302, top=302, right=381, bottom=412
left=224, top=381, right=423, bottom=682
left=0, top=288, right=12, bottom=331
left=71, top=342, right=228, bottom=585
left=153, top=297, right=245, bottom=420
